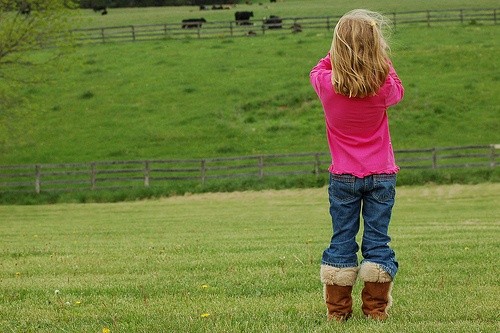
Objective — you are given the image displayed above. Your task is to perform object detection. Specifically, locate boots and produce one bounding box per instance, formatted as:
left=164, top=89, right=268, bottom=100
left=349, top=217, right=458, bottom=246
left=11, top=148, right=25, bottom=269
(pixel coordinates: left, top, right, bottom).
left=320, top=264, right=360, bottom=321
left=359, top=260, right=393, bottom=321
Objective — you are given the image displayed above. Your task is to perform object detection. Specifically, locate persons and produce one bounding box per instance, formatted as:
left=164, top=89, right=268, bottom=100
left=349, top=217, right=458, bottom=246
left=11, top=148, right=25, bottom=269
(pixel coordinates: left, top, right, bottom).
left=310, top=9, right=404, bottom=324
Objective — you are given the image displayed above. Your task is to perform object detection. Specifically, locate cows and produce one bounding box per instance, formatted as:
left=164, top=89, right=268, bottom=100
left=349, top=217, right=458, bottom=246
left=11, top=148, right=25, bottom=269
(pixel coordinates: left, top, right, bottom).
left=263, top=15, right=282, bottom=28
left=234, top=10, right=254, bottom=25
left=182, top=17, right=206, bottom=28
